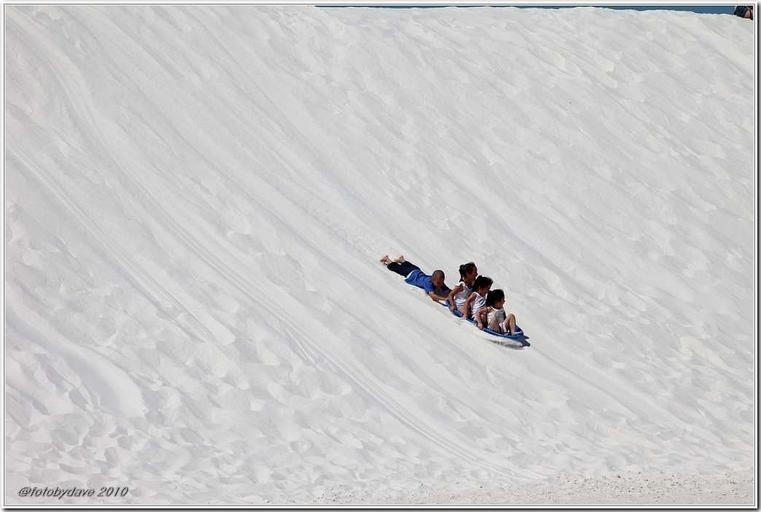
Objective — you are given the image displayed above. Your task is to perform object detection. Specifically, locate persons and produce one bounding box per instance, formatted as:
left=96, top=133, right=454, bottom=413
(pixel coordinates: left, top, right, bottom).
left=478, top=289, right=521, bottom=336
left=464, top=275, right=493, bottom=327
left=447, top=262, right=478, bottom=316
left=380, top=255, right=452, bottom=300
